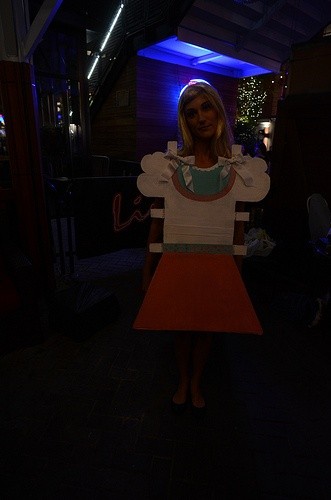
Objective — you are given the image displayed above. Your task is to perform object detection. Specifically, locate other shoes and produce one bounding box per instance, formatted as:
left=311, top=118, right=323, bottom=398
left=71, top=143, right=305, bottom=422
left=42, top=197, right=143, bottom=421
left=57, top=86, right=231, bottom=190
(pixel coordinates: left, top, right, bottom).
left=171, top=390, right=189, bottom=418
left=190, top=390, right=208, bottom=419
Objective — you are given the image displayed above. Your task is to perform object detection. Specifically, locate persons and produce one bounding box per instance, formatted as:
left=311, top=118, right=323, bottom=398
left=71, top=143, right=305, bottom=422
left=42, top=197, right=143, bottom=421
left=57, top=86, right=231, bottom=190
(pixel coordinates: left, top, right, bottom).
left=139, top=78, right=252, bottom=413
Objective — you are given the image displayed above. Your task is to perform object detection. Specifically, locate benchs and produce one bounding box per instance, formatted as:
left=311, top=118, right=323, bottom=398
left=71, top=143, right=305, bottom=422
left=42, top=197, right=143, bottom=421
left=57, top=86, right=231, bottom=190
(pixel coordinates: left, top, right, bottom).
left=44, top=172, right=161, bottom=329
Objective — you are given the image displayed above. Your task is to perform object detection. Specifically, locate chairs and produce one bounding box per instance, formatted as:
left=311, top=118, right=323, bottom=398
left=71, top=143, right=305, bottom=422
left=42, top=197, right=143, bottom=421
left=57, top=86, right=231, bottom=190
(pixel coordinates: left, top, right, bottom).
left=304, top=194, right=330, bottom=328
